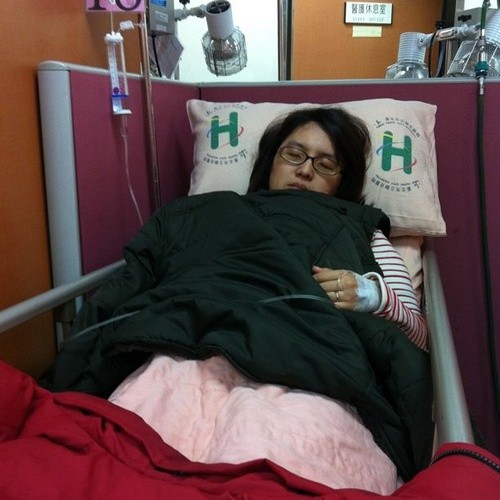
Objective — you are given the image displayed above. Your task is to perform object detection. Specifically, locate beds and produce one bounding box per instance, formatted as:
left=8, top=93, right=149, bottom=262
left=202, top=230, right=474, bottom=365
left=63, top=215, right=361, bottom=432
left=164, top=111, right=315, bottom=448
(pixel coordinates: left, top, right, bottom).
left=0, top=60, right=500, bottom=500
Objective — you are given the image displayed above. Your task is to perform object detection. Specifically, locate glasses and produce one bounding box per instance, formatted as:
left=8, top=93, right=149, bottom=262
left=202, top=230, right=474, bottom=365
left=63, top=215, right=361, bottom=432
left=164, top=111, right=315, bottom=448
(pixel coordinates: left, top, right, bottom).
left=277, top=144, right=343, bottom=176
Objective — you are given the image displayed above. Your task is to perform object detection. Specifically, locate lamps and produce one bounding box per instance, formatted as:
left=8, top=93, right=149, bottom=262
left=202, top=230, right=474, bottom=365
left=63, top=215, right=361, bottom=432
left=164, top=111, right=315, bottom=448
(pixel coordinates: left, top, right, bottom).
left=173, top=0, right=248, bottom=75
left=385, top=15, right=481, bottom=79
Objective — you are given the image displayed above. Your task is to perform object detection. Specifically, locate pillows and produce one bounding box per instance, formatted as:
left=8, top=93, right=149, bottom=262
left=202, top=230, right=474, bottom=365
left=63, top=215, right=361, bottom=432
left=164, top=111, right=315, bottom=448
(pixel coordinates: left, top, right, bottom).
left=185, top=98, right=446, bottom=238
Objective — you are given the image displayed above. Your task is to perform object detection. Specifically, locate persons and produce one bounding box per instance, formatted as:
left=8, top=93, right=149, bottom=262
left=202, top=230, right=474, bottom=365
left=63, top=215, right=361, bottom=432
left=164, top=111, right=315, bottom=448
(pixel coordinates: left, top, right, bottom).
left=246, top=109, right=428, bottom=352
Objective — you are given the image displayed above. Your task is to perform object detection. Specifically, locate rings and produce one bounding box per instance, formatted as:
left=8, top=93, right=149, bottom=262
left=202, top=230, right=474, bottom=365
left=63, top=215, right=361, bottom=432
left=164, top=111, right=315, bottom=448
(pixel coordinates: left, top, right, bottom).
left=335, top=292, right=340, bottom=301
left=337, top=278, right=344, bottom=291
